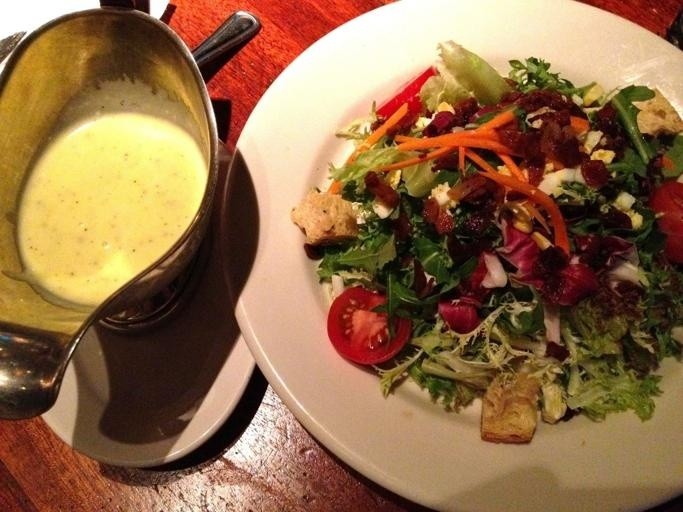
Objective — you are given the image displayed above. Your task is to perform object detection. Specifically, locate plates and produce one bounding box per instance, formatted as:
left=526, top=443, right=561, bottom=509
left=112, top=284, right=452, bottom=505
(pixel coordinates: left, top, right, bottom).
left=224, top=2, right=683, bottom=512
left=38, top=138, right=257, bottom=466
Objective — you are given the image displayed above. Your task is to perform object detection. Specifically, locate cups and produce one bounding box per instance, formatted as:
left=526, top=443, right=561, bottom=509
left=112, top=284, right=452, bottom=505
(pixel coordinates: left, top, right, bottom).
left=0, top=0, right=221, bottom=420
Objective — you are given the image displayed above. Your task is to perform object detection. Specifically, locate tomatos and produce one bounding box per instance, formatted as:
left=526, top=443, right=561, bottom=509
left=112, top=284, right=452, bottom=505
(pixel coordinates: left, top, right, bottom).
left=652, top=180, right=683, bottom=265
left=327, top=285, right=411, bottom=366
